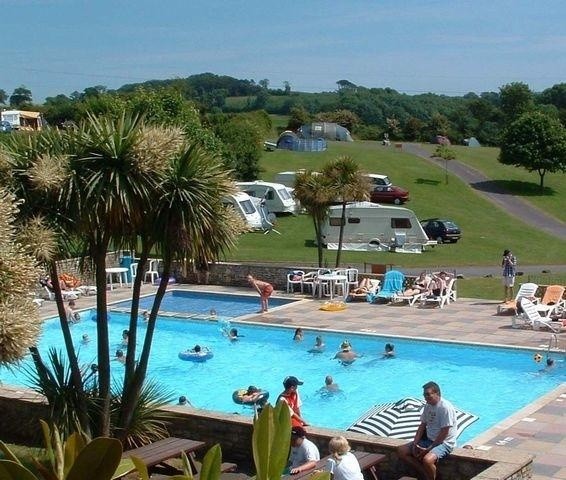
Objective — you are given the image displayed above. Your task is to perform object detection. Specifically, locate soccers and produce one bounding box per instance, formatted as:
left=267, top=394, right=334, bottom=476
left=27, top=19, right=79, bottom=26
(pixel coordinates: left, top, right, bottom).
left=534, top=355, right=542, bottom=362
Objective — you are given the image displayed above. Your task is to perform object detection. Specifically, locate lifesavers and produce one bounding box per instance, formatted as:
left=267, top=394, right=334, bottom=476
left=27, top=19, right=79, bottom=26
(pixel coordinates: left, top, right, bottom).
left=178, top=350, right=212, bottom=361
left=232, top=390, right=269, bottom=404
left=91, top=314, right=111, bottom=322
left=322, top=301, right=346, bottom=311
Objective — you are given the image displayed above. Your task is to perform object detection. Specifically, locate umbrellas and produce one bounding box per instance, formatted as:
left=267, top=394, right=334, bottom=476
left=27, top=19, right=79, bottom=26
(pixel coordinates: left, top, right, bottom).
left=346, top=396, right=480, bottom=445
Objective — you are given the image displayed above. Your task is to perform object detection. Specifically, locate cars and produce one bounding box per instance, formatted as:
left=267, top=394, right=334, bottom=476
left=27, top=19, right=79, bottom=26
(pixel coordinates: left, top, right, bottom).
left=0, top=120, right=11, bottom=134
left=252, top=142, right=277, bottom=153
left=367, top=185, right=411, bottom=206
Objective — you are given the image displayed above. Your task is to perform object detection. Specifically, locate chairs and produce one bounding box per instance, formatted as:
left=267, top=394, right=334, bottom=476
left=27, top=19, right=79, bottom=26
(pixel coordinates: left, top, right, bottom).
left=39, top=280, right=98, bottom=302
left=106, top=257, right=162, bottom=290
left=496, top=282, right=566, bottom=334
left=287, top=266, right=457, bottom=309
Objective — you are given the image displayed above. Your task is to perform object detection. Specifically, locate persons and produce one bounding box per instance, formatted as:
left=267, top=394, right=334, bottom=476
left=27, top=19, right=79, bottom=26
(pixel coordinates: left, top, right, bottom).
left=276, top=376, right=310, bottom=428
left=502, top=249, right=517, bottom=302
left=404, top=271, right=455, bottom=297
left=65, top=300, right=150, bottom=373
left=547, top=359, right=555, bottom=368
left=286, top=426, right=321, bottom=474
left=396, top=380, right=457, bottom=480
left=247, top=274, right=273, bottom=313
left=294, top=328, right=395, bottom=366
left=326, top=436, right=364, bottom=480
left=320, top=376, right=338, bottom=390
left=356, top=277, right=373, bottom=294
left=177, top=328, right=263, bottom=418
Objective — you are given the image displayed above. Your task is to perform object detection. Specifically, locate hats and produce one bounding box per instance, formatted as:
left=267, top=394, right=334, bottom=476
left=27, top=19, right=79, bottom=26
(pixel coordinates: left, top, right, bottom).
left=290, top=425, right=306, bottom=439
left=247, top=385, right=261, bottom=392
left=340, top=340, right=351, bottom=351
left=503, top=249, right=513, bottom=256
left=283, top=376, right=304, bottom=387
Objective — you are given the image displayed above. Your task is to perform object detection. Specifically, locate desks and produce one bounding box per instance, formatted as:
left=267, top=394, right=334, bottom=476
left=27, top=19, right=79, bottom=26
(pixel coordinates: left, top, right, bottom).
left=119, top=436, right=207, bottom=477
left=282, top=451, right=386, bottom=480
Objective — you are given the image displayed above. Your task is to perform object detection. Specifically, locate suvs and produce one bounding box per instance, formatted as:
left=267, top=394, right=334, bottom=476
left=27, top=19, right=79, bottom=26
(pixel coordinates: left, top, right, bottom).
left=359, top=173, right=393, bottom=192
left=420, top=219, right=462, bottom=245
